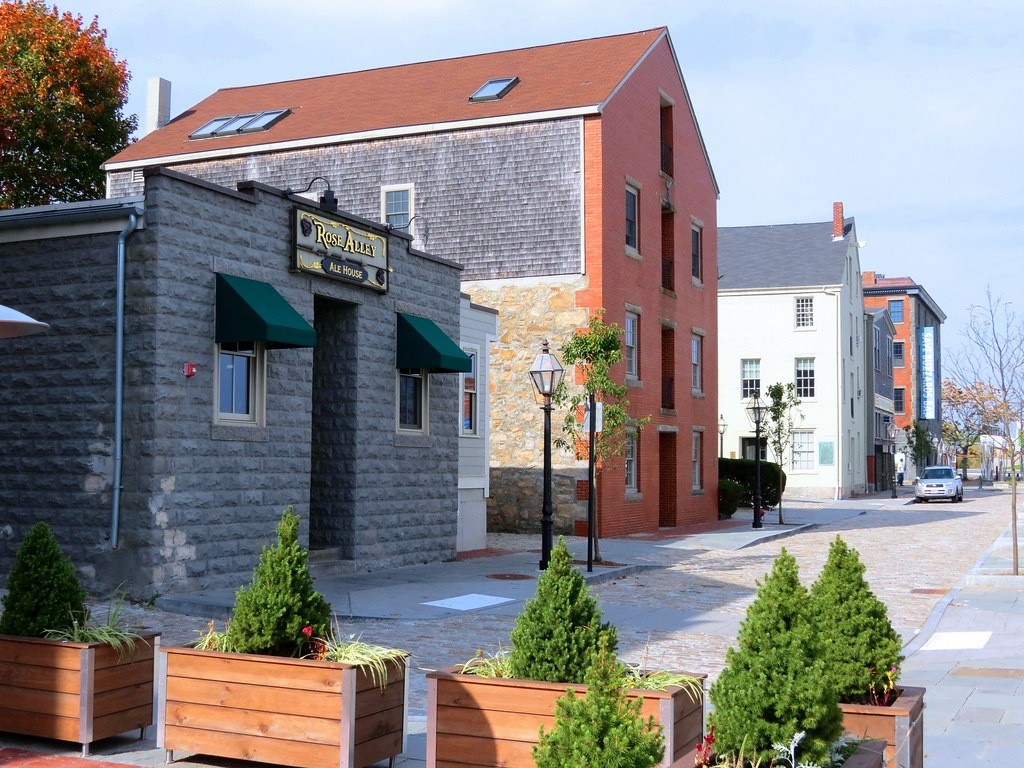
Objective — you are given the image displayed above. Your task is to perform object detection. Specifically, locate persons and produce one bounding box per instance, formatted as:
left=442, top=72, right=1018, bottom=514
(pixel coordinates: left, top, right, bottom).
left=898, top=458, right=905, bottom=486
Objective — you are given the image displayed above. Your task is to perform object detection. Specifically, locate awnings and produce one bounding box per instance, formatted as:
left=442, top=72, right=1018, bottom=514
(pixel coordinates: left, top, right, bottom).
left=219, top=270, right=313, bottom=354
left=393, top=314, right=474, bottom=375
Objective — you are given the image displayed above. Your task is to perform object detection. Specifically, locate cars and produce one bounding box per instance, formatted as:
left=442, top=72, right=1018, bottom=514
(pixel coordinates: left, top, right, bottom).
left=913, top=465, right=964, bottom=503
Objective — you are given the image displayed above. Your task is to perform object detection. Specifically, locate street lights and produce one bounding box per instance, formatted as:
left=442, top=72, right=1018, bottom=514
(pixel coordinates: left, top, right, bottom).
left=718, top=413, right=727, bottom=458
left=529, top=338, right=567, bottom=570
left=746, top=386, right=762, bottom=528
left=889, top=422, right=898, bottom=498
